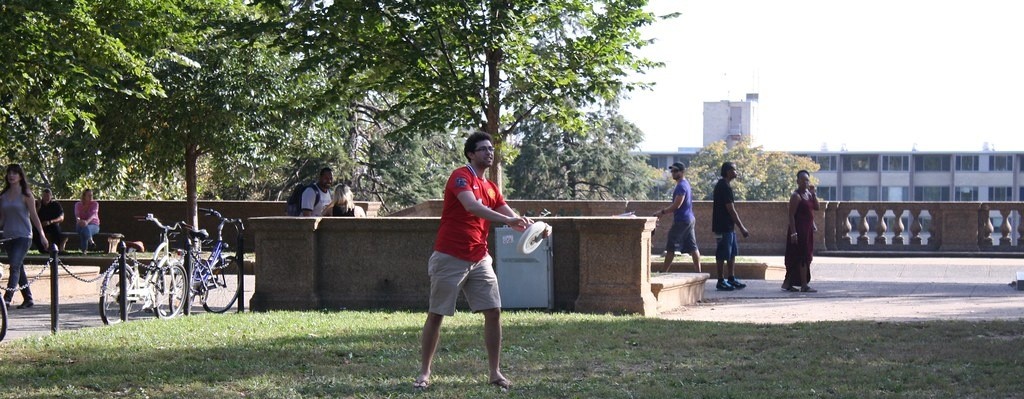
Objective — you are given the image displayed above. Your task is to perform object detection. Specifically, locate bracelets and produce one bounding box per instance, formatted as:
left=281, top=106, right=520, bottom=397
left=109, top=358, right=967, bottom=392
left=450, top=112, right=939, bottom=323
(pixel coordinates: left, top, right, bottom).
left=791, top=233, right=797, bottom=237
left=661, top=209, right=665, bottom=215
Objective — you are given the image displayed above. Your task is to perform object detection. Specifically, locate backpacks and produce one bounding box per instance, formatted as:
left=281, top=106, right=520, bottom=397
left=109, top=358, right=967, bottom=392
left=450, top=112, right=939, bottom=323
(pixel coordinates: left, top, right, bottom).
left=286, top=183, right=320, bottom=216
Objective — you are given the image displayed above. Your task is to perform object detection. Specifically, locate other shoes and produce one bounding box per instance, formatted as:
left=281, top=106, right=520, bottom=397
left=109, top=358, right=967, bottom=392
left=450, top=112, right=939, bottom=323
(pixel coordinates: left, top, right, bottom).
left=90, top=243, right=97, bottom=250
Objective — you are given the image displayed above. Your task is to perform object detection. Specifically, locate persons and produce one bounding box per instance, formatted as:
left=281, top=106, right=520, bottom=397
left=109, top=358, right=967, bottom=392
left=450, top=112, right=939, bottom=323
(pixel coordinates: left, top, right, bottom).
left=32, top=188, right=65, bottom=255
left=0, top=163, right=49, bottom=311
left=414, top=132, right=550, bottom=390
left=781, top=169, right=820, bottom=292
left=74, top=188, right=101, bottom=256
left=653, top=162, right=702, bottom=274
left=323, top=183, right=366, bottom=218
left=298, top=167, right=335, bottom=218
left=710, top=161, right=750, bottom=292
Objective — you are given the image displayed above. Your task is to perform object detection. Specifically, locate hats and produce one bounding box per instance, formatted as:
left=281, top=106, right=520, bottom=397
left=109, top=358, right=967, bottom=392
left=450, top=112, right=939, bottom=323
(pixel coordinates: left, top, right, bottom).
left=668, top=162, right=686, bottom=171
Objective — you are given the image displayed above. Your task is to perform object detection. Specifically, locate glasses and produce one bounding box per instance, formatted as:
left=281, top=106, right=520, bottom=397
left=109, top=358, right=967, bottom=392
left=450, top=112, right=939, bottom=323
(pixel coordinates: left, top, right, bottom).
left=670, top=169, right=681, bottom=173
left=474, top=147, right=495, bottom=152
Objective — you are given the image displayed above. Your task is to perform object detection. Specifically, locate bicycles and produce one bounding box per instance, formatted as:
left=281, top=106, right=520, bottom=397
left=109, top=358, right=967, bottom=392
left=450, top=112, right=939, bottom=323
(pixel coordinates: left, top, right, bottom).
left=98, top=213, right=195, bottom=325
left=169, top=207, right=246, bottom=316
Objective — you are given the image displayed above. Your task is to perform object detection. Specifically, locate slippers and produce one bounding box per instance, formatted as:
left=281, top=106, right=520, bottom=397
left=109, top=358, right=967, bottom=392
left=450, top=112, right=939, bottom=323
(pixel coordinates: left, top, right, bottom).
left=800, top=285, right=817, bottom=292
left=781, top=285, right=799, bottom=292
left=413, top=378, right=429, bottom=390
left=491, top=376, right=514, bottom=391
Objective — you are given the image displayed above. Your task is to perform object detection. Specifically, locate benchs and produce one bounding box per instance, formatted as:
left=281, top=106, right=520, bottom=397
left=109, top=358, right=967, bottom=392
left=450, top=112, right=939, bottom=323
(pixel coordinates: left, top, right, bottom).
left=60, top=232, right=125, bottom=254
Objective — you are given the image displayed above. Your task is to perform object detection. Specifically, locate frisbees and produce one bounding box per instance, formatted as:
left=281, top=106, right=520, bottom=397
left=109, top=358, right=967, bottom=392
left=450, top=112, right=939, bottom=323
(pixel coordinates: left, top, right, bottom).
left=517, top=221, right=551, bottom=256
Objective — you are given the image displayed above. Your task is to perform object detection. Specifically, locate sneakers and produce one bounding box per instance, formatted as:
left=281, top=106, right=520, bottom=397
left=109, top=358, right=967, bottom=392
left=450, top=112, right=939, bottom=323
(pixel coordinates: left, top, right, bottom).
left=16, top=300, right=34, bottom=310
left=727, top=280, right=746, bottom=290
left=716, top=280, right=736, bottom=290
